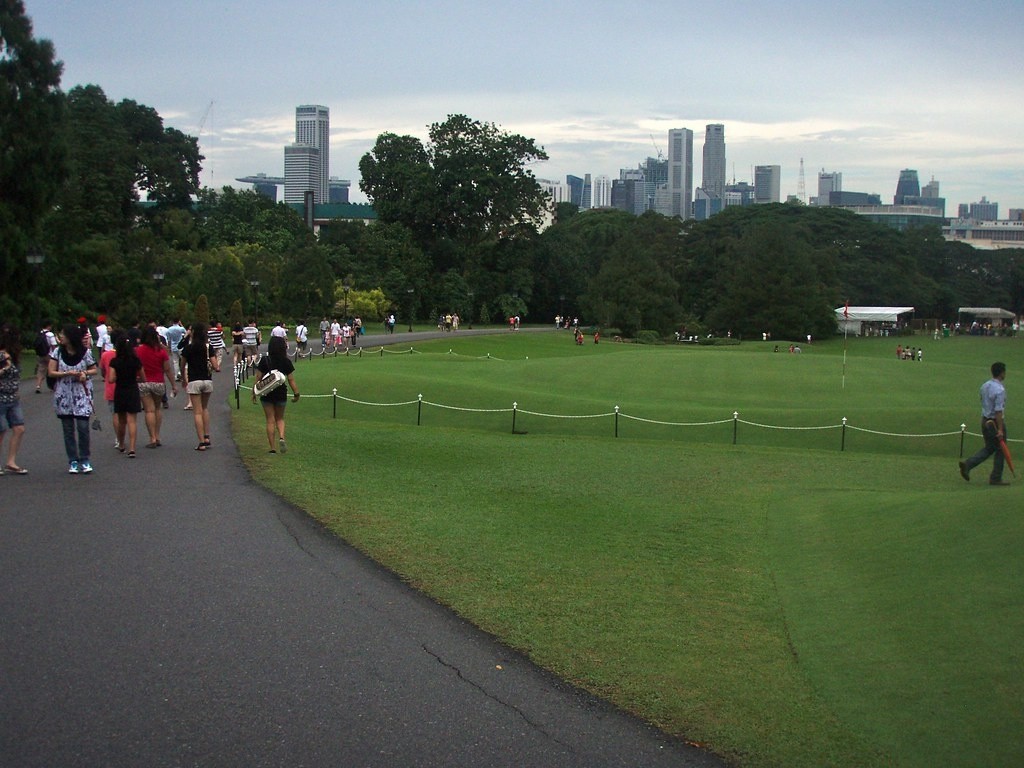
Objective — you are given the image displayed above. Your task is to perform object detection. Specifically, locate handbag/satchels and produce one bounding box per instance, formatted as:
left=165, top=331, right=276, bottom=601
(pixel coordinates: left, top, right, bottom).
left=45, top=345, right=62, bottom=390
left=296, top=336, right=302, bottom=344
left=254, top=356, right=286, bottom=397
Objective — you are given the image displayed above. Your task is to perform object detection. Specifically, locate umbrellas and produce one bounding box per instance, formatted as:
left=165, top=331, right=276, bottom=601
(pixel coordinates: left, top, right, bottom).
left=985, top=419, right=1016, bottom=478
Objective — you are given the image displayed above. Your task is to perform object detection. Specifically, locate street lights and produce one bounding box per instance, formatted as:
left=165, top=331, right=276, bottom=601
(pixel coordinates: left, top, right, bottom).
left=513, top=291, right=518, bottom=329
left=468, top=291, right=473, bottom=329
left=25, top=247, right=45, bottom=331
left=342, top=282, right=350, bottom=322
left=153, top=266, right=165, bottom=326
left=560, top=295, right=565, bottom=316
left=407, top=285, right=413, bottom=332
left=250, top=274, right=259, bottom=328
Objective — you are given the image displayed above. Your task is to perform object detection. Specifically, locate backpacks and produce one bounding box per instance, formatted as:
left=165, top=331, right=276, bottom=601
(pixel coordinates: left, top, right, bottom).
left=34, top=331, right=49, bottom=357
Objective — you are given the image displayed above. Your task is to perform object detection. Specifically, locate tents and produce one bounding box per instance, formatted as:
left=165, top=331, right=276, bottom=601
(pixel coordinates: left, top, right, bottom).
left=834, top=307, right=915, bottom=335
left=958, top=308, right=1015, bottom=325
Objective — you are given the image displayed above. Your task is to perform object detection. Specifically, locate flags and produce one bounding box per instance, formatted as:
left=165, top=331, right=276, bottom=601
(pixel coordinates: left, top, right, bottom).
left=844, top=301, right=848, bottom=318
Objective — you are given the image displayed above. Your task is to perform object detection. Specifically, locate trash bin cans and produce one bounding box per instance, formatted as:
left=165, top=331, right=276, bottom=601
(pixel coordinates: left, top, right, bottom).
left=360, top=326, right=366, bottom=335
left=942, top=329, right=950, bottom=337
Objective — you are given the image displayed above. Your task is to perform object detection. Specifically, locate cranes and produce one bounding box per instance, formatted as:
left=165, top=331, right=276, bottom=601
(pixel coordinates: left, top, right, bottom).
left=651, top=133, right=663, bottom=158
left=194, top=100, right=213, bottom=136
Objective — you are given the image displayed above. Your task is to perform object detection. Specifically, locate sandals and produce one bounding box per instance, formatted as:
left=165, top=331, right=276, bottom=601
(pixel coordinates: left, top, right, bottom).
left=194, top=442, right=205, bottom=451
left=204, top=435, right=212, bottom=448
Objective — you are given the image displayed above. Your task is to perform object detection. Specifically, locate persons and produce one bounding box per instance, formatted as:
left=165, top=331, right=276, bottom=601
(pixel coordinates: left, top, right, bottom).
left=896, top=344, right=923, bottom=361
left=36, top=319, right=309, bottom=473
left=319, top=315, right=362, bottom=349
left=932, top=315, right=1024, bottom=340
left=0, top=349, right=28, bottom=475
left=959, top=362, right=1010, bottom=485
left=509, top=313, right=520, bottom=331
left=438, top=312, right=459, bottom=331
left=385, top=315, right=395, bottom=335
left=862, top=321, right=909, bottom=337
left=677, top=329, right=811, bottom=354
left=555, top=314, right=599, bottom=345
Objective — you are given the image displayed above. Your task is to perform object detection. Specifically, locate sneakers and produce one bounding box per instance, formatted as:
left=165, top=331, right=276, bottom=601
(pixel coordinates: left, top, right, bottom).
left=79, top=462, right=93, bottom=473
left=69, top=460, right=79, bottom=474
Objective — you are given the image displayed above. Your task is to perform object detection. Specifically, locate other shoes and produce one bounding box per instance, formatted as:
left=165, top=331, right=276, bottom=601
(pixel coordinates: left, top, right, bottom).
left=269, top=449, right=276, bottom=455
left=36, top=385, right=41, bottom=393
left=146, top=442, right=157, bottom=448
left=4, top=464, right=28, bottom=473
left=163, top=400, right=169, bottom=408
left=127, top=450, right=136, bottom=457
left=156, top=440, right=162, bottom=446
left=175, top=371, right=183, bottom=382
left=116, top=446, right=127, bottom=453
left=0, top=466, right=4, bottom=475
left=959, top=461, right=970, bottom=481
left=184, top=405, right=193, bottom=410
left=279, top=438, right=286, bottom=453
left=989, top=478, right=1010, bottom=485
